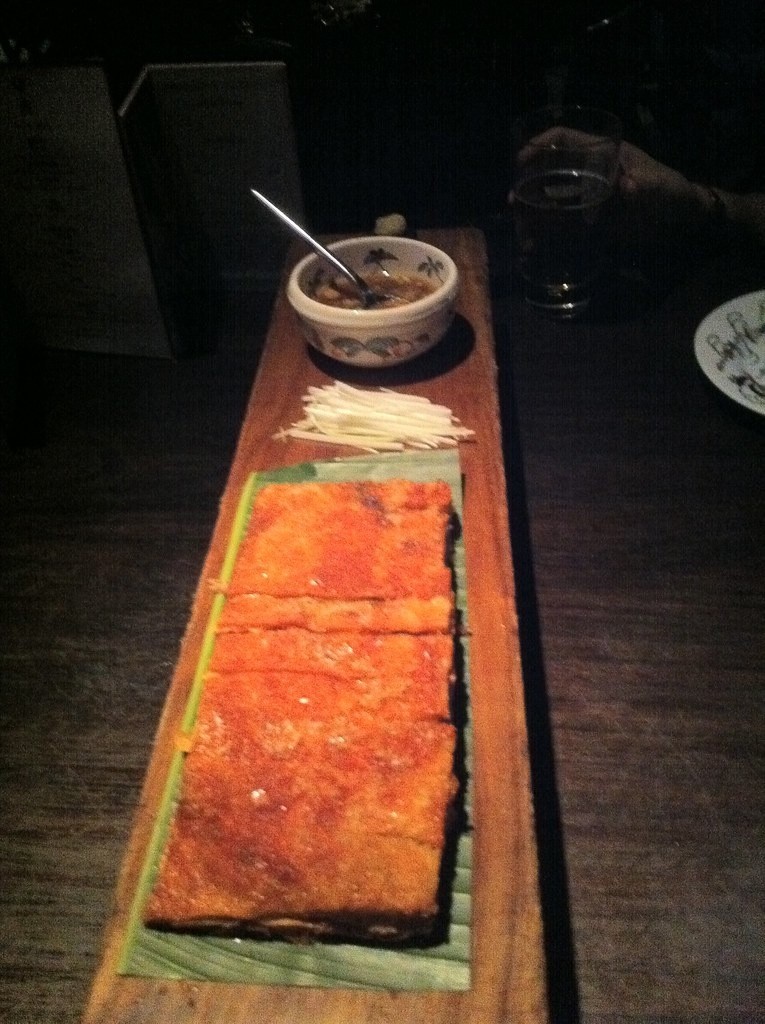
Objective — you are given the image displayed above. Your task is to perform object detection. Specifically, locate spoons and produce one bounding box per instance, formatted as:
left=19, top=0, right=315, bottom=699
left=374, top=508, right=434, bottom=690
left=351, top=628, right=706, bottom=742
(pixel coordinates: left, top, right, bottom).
left=251, top=188, right=412, bottom=310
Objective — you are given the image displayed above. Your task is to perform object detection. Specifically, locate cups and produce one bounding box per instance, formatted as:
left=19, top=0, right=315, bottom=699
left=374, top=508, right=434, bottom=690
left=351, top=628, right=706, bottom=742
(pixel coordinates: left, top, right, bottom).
left=510, top=105, right=624, bottom=318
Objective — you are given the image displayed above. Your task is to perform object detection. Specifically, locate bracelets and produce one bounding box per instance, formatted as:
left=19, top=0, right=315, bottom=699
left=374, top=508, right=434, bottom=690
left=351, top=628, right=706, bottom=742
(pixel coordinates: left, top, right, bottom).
left=709, top=187, right=727, bottom=233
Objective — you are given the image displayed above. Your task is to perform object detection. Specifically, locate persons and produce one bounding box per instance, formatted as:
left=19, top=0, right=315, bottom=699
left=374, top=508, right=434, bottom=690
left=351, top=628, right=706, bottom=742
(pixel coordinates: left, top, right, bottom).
left=504, top=126, right=764, bottom=254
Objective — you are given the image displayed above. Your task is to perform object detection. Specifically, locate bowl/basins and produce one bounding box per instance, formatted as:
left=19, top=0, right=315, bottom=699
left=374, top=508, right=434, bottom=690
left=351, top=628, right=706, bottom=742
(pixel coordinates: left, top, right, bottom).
left=286, top=236, right=460, bottom=367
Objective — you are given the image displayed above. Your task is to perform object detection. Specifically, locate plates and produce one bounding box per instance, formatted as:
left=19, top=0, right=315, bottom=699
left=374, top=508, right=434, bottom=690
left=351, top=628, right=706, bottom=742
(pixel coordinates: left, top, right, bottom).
left=694, top=291, right=765, bottom=415
